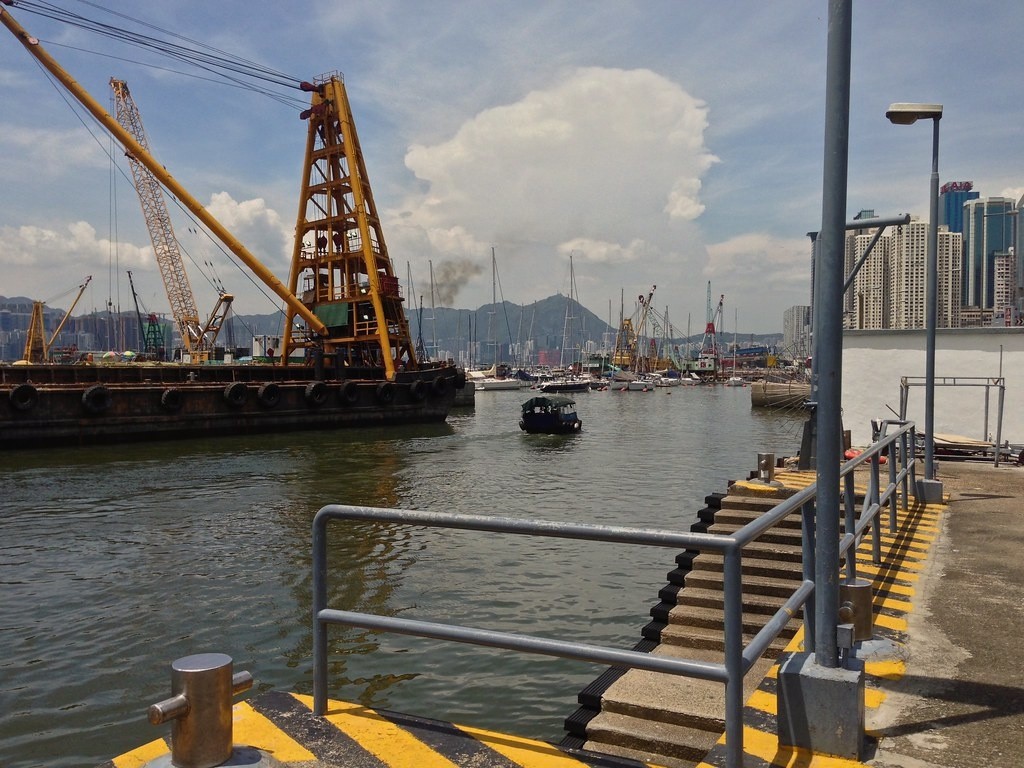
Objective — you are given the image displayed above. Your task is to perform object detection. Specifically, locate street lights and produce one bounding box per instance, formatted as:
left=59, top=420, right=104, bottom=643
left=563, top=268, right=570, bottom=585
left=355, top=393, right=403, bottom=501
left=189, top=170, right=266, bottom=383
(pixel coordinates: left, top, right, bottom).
left=884, top=102, right=970, bottom=505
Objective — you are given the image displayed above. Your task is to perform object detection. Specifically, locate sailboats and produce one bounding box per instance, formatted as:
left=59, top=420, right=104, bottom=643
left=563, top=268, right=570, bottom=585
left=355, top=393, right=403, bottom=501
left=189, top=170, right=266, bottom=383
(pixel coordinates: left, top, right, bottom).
left=406, top=246, right=749, bottom=394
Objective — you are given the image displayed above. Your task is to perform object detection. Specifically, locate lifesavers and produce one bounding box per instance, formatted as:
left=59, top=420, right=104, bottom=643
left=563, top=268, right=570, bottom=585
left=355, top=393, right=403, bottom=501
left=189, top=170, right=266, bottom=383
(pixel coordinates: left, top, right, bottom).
left=457, top=374, right=465, bottom=389
left=845, top=449, right=887, bottom=464
left=305, top=381, right=328, bottom=406
left=161, top=388, right=184, bottom=412
left=432, top=375, right=447, bottom=396
left=9, top=383, right=39, bottom=410
left=224, top=382, right=247, bottom=409
left=410, top=380, right=426, bottom=400
left=570, top=422, right=577, bottom=433
left=519, top=421, right=525, bottom=430
left=81, top=384, right=112, bottom=413
left=341, top=381, right=360, bottom=405
left=377, top=382, right=394, bottom=402
left=559, top=421, right=570, bottom=435
left=257, top=382, right=280, bottom=408
left=578, top=420, right=582, bottom=431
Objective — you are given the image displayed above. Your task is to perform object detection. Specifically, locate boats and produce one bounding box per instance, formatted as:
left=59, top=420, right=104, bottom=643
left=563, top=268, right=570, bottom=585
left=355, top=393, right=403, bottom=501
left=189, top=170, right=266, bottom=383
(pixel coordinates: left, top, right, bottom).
left=1, top=0, right=467, bottom=450
left=519, top=395, right=590, bottom=435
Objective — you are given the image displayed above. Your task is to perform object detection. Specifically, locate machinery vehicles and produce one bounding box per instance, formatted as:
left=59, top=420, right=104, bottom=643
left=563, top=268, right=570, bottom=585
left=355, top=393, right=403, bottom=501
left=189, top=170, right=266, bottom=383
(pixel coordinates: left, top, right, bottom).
left=102, top=73, right=240, bottom=365
